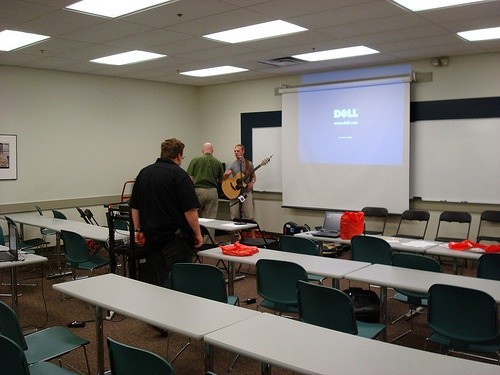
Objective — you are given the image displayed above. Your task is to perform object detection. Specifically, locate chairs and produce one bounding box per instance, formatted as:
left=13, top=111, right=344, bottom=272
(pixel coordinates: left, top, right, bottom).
left=0, top=207, right=500, bottom=375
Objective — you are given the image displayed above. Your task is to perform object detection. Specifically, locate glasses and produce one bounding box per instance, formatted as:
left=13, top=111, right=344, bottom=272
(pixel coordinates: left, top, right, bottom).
left=181, top=155, right=184, bottom=159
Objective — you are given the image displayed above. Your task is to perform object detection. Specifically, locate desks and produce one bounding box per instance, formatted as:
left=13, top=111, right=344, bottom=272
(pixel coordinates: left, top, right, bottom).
left=218, top=199, right=240, bottom=219
left=293, top=230, right=443, bottom=321
left=197, top=244, right=371, bottom=296
left=426, top=242, right=483, bottom=260
left=344, top=263, right=500, bottom=343
left=199, top=218, right=257, bottom=283
left=0, top=214, right=72, bottom=279
left=1, top=245, right=47, bottom=335
left=205, top=312, right=500, bottom=375
left=46, top=221, right=136, bottom=276
left=53, top=273, right=261, bottom=375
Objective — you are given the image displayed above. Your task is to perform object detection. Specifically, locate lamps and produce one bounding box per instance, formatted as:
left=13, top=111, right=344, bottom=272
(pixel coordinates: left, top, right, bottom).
left=431, top=57, right=449, bottom=67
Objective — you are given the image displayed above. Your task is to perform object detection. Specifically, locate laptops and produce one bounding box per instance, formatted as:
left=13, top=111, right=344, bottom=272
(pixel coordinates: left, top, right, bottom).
left=312, top=211, right=346, bottom=238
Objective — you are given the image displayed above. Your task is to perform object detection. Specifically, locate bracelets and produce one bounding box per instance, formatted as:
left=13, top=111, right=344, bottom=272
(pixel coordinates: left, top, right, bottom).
left=252, top=181, right=254, bottom=183
left=135, top=227, right=141, bottom=232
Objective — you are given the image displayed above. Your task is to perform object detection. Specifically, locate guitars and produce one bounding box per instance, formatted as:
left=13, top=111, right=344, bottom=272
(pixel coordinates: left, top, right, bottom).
left=219, top=156, right=270, bottom=200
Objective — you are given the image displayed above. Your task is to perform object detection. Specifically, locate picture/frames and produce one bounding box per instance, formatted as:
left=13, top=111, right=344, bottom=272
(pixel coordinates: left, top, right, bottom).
left=0, top=134, right=18, bottom=180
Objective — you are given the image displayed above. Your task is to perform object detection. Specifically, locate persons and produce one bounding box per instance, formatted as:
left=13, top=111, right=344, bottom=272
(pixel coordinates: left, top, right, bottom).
left=187, top=143, right=224, bottom=244
left=224, top=144, right=256, bottom=240
left=129, top=138, right=203, bottom=290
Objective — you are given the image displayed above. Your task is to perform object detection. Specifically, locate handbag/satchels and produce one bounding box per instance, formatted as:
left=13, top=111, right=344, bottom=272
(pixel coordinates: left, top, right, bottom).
left=339, top=211, right=365, bottom=238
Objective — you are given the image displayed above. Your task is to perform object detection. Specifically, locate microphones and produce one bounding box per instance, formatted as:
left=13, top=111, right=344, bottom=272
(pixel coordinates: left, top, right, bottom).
left=230, top=194, right=248, bottom=208
